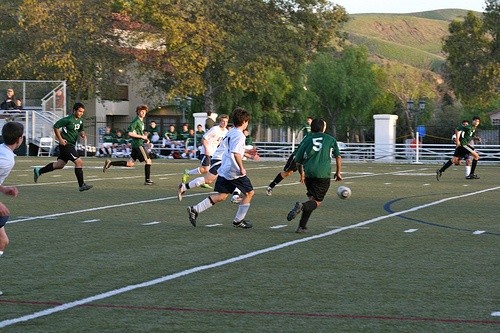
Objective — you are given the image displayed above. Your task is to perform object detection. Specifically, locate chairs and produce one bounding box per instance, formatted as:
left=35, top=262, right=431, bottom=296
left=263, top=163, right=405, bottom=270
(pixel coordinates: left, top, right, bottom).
left=37, top=137, right=53, bottom=157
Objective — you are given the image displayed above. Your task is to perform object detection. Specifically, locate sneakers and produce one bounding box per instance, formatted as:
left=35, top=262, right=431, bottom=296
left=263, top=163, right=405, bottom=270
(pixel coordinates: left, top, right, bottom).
left=186, top=205, right=198, bottom=227
left=232, top=219, right=253, bottom=229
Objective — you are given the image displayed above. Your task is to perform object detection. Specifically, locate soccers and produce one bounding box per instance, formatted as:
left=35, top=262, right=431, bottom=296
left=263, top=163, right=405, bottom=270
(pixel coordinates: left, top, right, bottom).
left=336, top=186, right=351, bottom=199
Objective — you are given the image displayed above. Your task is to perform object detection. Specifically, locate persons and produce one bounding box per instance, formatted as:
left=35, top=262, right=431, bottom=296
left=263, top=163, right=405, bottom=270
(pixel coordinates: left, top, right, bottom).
left=0, top=87, right=23, bottom=121
left=0, top=121, right=24, bottom=294
left=34, top=102, right=93, bottom=192
left=265, top=119, right=326, bottom=195
left=436, top=115, right=481, bottom=181
left=182, top=114, right=229, bottom=189
left=286, top=117, right=342, bottom=233
left=46, top=90, right=64, bottom=111
left=408, top=135, right=421, bottom=164
left=295, top=116, right=313, bottom=159
left=103, top=103, right=155, bottom=184
left=178, top=130, right=260, bottom=201
left=187, top=108, right=257, bottom=228
left=100, top=110, right=254, bottom=160
left=451, top=119, right=476, bottom=178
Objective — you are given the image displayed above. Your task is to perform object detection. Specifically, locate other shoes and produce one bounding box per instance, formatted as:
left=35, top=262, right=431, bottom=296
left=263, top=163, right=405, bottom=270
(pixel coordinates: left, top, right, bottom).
left=266, top=187, right=273, bottom=196
left=297, top=227, right=311, bottom=233
left=177, top=182, right=185, bottom=202
left=286, top=202, right=302, bottom=221
left=465, top=174, right=477, bottom=179
left=232, top=196, right=243, bottom=204
left=199, top=184, right=213, bottom=189
left=469, top=174, right=480, bottom=180
left=144, top=180, right=154, bottom=185
left=79, top=183, right=93, bottom=192
left=181, top=168, right=189, bottom=184
left=102, top=159, right=111, bottom=173
left=32, top=168, right=40, bottom=183
left=436, top=168, right=442, bottom=181
